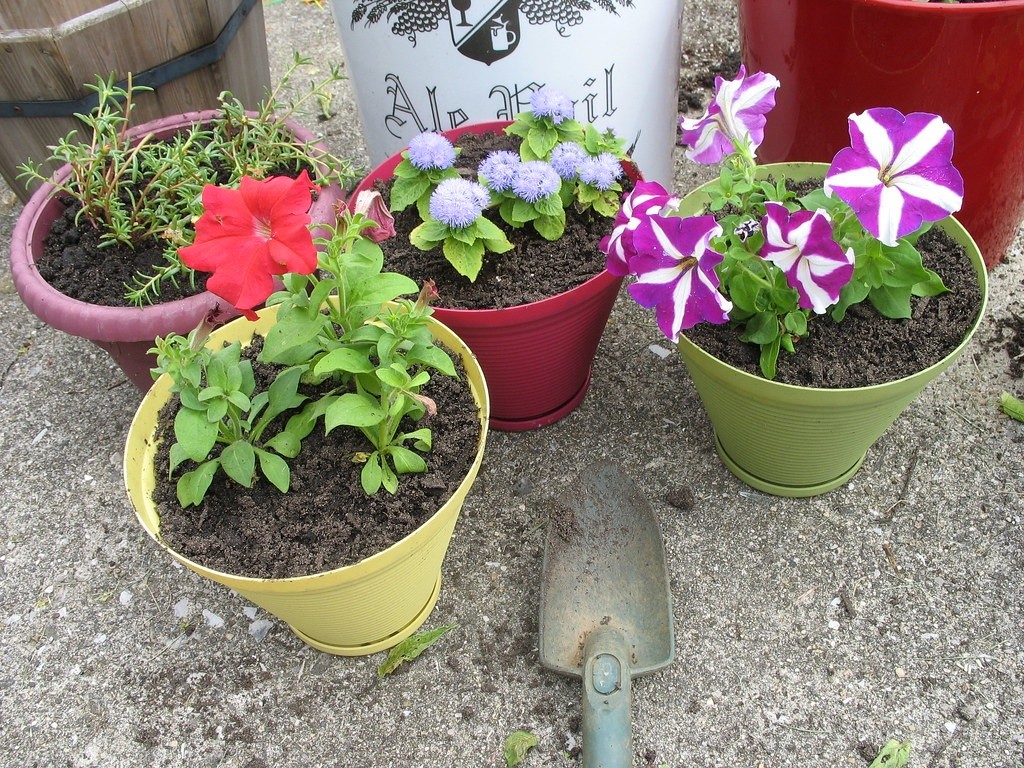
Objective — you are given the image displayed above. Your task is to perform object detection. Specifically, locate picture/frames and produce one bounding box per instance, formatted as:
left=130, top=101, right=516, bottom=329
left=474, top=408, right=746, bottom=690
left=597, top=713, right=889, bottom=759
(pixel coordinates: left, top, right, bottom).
left=15, top=49, right=350, bottom=322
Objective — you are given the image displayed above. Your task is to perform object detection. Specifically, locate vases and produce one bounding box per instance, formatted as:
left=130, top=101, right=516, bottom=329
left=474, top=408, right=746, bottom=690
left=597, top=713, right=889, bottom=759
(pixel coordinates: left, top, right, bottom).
left=338, top=120, right=644, bottom=431
left=10, top=109, right=345, bottom=394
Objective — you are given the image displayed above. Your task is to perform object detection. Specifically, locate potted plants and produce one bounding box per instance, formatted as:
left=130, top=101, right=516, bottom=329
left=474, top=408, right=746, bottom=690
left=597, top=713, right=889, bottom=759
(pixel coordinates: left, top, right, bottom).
left=123, top=209, right=490, bottom=657
left=665, top=161, right=989, bottom=497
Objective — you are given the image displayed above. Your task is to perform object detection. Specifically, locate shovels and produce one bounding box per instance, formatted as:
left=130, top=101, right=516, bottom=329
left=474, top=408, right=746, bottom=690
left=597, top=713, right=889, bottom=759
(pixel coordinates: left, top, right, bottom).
left=538, top=456, right=677, bottom=768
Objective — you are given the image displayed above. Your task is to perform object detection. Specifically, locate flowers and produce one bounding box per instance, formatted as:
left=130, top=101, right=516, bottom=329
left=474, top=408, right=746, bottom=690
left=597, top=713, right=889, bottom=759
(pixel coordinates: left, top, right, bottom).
left=597, top=64, right=965, bottom=372
left=388, top=90, right=622, bottom=284
